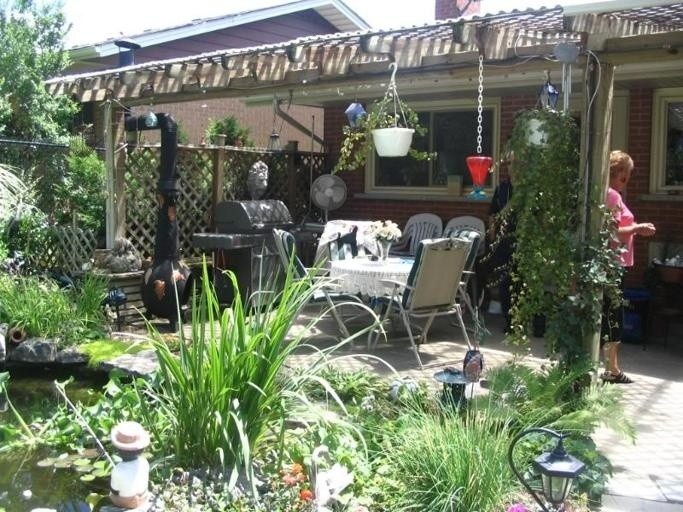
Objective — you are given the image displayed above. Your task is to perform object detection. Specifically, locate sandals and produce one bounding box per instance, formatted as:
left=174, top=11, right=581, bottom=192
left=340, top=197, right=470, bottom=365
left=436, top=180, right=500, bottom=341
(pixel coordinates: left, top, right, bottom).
left=603, top=369, right=635, bottom=384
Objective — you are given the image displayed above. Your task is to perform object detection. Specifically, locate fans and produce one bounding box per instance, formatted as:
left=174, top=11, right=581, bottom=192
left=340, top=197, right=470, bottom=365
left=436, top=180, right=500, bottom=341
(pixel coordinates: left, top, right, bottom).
left=308, top=173, right=348, bottom=224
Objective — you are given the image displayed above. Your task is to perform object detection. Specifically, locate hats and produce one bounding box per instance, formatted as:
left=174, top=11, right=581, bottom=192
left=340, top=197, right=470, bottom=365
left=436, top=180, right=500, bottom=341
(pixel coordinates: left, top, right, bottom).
left=109, top=421, right=150, bottom=452
left=499, top=150, right=514, bottom=163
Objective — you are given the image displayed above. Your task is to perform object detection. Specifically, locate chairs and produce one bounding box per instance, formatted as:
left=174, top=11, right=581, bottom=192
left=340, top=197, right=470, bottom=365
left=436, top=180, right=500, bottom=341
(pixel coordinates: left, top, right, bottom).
left=373, top=236, right=475, bottom=370
left=454, top=228, right=489, bottom=339
left=326, top=218, right=387, bottom=340
left=271, top=227, right=365, bottom=348
left=440, top=216, right=487, bottom=350
left=384, top=213, right=442, bottom=257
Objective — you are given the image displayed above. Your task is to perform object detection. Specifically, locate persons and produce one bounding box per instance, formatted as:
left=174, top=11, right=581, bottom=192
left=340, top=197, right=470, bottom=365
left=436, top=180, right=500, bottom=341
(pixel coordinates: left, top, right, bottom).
left=599, top=150, right=656, bottom=383
left=108, top=421, right=150, bottom=508
left=489, top=151, right=521, bottom=334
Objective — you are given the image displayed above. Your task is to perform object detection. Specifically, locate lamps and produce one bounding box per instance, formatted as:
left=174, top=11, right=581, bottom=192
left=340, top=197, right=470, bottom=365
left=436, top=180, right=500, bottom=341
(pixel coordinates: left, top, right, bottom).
left=505, top=426, right=588, bottom=512
left=143, top=89, right=159, bottom=129
left=344, top=84, right=369, bottom=132
left=264, top=99, right=285, bottom=153
left=536, top=67, right=559, bottom=113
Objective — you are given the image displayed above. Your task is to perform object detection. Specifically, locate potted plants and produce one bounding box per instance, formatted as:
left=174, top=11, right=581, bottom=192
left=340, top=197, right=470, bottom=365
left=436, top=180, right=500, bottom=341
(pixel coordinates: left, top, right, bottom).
left=329, top=90, right=439, bottom=174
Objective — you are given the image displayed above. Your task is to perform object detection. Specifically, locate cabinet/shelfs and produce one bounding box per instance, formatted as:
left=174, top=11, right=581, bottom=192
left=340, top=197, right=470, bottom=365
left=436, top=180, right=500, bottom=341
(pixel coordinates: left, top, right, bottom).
left=640, top=284, right=682, bottom=354
